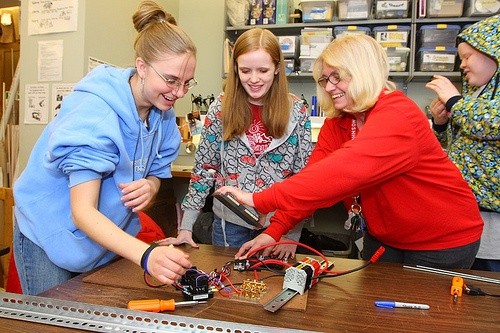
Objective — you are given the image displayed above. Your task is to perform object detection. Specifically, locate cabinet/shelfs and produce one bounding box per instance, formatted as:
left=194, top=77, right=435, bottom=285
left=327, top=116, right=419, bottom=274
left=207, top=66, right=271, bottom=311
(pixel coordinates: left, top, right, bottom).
left=222, top=0, right=414, bottom=85
left=408, top=0, right=500, bottom=86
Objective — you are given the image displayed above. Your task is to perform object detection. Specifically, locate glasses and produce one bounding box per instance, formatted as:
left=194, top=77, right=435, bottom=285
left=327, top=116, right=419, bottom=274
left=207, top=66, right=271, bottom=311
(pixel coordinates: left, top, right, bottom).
left=318, top=71, right=353, bottom=87
left=144, top=60, right=199, bottom=90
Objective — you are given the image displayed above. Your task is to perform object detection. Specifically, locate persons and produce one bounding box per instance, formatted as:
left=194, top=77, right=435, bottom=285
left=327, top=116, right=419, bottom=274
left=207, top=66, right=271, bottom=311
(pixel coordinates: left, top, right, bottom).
left=12, top=0, right=198, bottom=295
left=215, top=32, right=484, bottom=273
left=157, top=27, right=312, bottom=259
left=425, top=14, right=500, bottom=273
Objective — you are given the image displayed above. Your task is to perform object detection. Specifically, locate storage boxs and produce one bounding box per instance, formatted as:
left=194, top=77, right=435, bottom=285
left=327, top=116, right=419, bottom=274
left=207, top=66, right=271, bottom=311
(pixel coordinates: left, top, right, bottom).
left=225, top=0, right=500, bottom=76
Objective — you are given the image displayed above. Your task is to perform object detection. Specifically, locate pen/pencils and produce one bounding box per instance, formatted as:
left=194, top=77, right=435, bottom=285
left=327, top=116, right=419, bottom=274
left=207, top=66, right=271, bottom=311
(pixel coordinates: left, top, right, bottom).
left=374, top=300, right=430, bottom=310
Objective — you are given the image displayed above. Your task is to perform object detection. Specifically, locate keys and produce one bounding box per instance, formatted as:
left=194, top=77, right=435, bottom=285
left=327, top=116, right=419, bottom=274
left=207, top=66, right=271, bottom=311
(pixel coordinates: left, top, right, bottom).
left=344, top=207, right=367, bottom=233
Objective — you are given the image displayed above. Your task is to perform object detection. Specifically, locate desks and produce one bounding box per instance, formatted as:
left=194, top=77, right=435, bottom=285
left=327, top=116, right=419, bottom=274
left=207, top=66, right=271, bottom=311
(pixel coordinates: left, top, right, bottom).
left=164, top=155, right=352, bottom=256
left=0, top=241, right=500, bottom=333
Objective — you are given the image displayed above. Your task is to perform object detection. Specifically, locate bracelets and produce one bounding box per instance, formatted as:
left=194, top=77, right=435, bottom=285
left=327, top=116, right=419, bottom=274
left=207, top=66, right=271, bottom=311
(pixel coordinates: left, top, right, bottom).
left=140, top=243, right=156, bottom=275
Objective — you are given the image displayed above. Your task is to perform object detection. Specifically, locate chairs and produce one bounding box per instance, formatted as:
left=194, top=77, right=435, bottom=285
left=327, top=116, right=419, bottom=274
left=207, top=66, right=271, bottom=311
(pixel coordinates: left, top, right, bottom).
left=1, top=186, right=15, bottom=292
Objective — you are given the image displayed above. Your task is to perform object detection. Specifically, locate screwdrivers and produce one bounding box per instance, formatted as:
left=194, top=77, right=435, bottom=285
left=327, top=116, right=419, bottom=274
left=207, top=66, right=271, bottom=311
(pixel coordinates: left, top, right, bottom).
left=128, top=299, right=198, bottom=312
